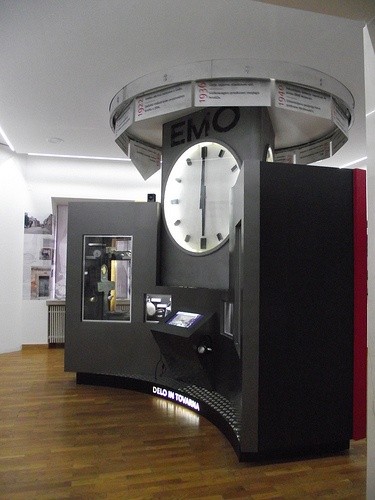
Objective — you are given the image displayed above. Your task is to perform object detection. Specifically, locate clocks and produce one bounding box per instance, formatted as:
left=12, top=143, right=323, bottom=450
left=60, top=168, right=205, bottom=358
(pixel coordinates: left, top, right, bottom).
left=162, top=138, right=245, bottom=257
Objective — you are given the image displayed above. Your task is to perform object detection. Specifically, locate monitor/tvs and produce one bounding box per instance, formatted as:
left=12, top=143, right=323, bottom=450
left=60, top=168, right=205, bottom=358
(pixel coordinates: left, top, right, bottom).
left=166, top=310, right=201, bottom=329
